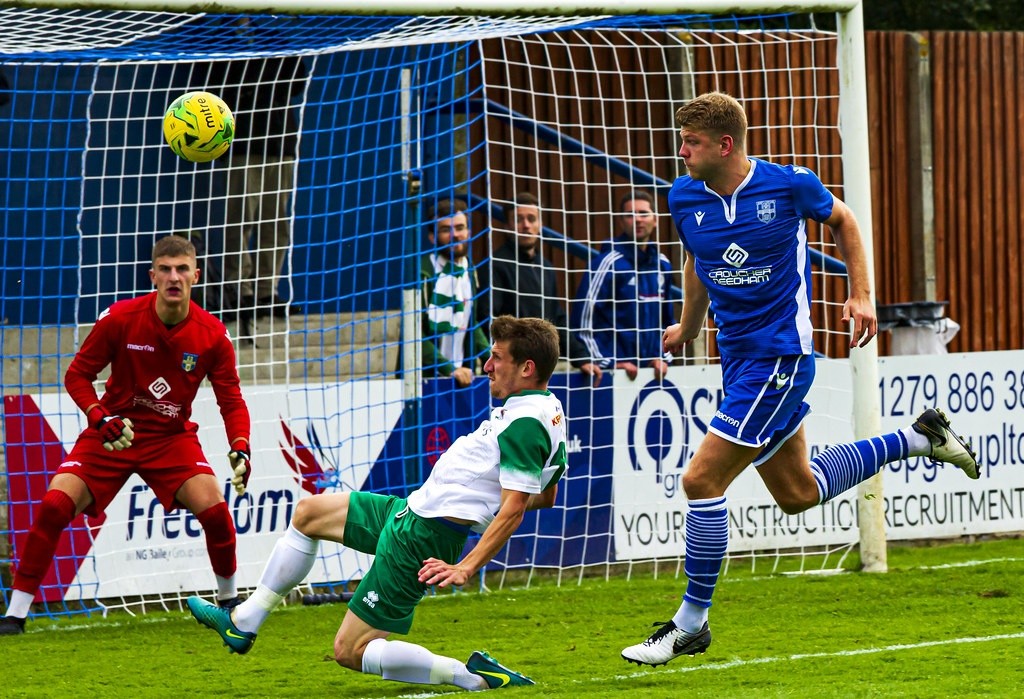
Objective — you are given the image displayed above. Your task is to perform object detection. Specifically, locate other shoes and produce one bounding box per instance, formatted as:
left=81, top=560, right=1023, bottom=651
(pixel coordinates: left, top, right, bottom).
left=230, top=296, right=263, bottom=318
left=220, top=596, right=247, bottom=610
left=0, top=614, right=27, bottom=636
left=260, top=296, right=302, bottom=317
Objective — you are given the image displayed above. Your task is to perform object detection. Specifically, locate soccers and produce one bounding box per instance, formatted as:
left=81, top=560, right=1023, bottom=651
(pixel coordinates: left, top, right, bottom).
left=161, top=91, right=237, bottom=164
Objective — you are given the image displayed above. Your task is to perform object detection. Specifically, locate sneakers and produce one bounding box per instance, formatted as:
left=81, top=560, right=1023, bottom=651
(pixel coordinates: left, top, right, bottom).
left=621, top=619, right=711, bottom=668
left=466, top=648, right=536, bottom=689
left=912, top=408, right=981, bottom=479
left=186, top=594, right=257, bottom=655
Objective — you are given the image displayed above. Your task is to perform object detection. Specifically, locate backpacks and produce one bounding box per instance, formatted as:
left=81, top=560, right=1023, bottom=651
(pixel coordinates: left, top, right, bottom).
left=185, top=231, right=237, bottom=322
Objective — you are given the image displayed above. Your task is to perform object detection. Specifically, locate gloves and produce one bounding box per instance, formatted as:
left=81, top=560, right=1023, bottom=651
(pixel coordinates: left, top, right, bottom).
left=96, top=414, right=135, bottom=451
left=228, top=450, right=251, bottom=496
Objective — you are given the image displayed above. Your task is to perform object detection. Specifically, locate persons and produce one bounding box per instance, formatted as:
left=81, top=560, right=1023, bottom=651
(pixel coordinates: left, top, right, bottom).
left=188, top=315, right=570, bottom=690
left=1, top=236, right=252, bottom=634
left=620, top=94, right=982, bottom=667
left=574, top=189, right=674, bottom=381
left=477, top=190, right=605, bottom=386
left=397, top=202, right=492, bottom=383
